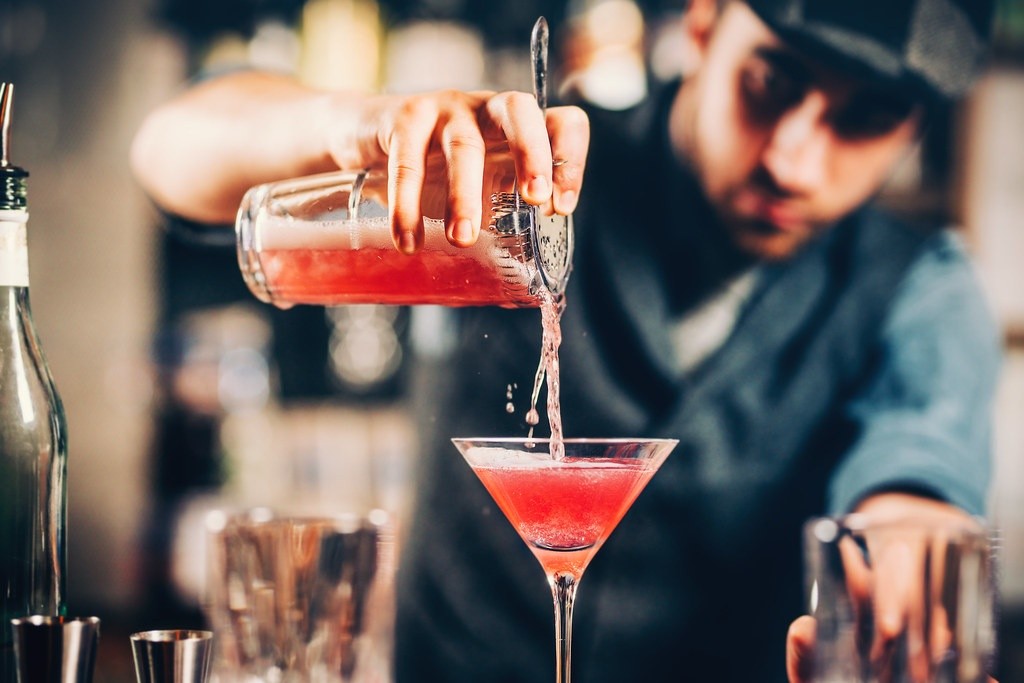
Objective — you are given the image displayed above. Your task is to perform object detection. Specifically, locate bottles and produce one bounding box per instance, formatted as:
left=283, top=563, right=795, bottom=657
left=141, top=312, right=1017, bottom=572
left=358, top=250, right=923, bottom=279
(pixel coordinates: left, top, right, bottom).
left=0, top=164, right=67, bottom=683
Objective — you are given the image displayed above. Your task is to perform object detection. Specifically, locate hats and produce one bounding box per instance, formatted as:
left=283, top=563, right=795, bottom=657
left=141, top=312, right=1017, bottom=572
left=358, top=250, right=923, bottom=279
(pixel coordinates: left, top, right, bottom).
left=743, top=0, right=982, bottom=115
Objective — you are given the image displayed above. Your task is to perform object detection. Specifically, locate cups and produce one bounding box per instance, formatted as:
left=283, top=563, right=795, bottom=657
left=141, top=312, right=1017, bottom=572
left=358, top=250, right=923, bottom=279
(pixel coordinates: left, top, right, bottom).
left=801, top=516, right=1002, bottom=683
left=130, top=629, right=212, bottom=683
left=235, top=143, right=574, bottom=309
left=198, top=503, right=389, bottom=683
left=10, top=616, right=101, bottom=683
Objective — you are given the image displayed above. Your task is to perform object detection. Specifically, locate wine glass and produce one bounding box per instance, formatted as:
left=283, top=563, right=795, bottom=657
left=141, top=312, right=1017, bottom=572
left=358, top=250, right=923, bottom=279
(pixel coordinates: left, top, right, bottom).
left=450, top=436, right=681, bottom=683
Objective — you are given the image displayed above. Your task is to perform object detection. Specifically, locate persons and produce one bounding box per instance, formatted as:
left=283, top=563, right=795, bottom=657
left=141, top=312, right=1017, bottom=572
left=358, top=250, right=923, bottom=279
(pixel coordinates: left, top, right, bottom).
left=129, top=0, right=1000, bottom=683
